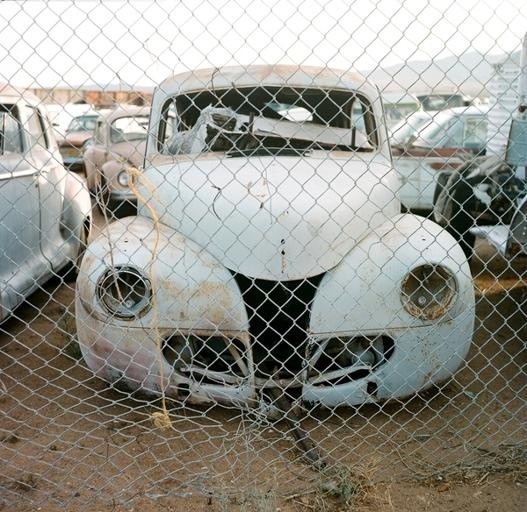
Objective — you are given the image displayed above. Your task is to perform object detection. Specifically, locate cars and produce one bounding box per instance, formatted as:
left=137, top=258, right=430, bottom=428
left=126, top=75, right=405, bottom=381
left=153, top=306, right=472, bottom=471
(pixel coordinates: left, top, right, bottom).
left=46, top=103, right=176, bottom=215
left=381, top=83, right=526, bottom=257
left=73, top=64, right=475, bottom=421
left=1, top=95, right=91, bottom=322
